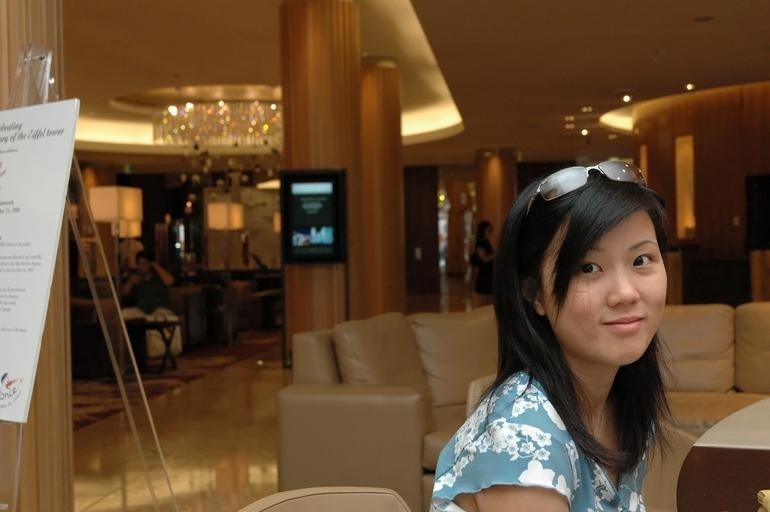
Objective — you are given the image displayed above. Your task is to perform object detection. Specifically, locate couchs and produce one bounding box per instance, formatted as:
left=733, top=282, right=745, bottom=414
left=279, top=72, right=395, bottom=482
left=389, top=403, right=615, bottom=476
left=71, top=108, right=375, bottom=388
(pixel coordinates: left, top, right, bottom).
left=71, top=276, right=279, bottom=348
left=276, top=330, right=770, bottom=512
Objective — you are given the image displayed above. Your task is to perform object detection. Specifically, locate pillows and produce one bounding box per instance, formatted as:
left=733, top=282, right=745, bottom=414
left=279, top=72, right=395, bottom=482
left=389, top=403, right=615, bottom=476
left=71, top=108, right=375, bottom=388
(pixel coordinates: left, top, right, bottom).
left=735, top=303, right=768, bottom=396
left=333, top=316, right=430, bottom=412
left=652, top=307, right=734, bottom=391
left=412, top=311, right=505, bottom=403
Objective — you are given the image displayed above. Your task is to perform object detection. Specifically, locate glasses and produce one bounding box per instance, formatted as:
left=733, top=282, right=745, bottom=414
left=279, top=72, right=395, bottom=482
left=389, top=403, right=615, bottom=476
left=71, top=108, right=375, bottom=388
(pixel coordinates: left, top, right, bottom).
left=526, top=159, right=648, bottom=217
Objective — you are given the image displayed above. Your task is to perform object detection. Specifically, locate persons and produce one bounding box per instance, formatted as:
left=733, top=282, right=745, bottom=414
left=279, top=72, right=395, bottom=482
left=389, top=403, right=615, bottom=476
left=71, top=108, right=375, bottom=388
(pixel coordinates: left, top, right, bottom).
left=427, top=161, right=674, bottom=512
left=119, top=251, right=175, bottom=373
left=474, top=219, right=496, bottom=308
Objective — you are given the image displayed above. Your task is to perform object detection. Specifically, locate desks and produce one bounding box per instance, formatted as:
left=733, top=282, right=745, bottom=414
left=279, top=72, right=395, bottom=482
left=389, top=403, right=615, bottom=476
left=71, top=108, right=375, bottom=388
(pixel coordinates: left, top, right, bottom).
left=74, top=320, right=180, bottom=377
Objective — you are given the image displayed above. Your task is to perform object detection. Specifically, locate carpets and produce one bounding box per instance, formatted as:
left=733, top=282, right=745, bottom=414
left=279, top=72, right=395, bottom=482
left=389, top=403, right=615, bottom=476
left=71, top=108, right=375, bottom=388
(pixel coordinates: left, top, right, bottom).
left=73, top=339, right=278, bottom=432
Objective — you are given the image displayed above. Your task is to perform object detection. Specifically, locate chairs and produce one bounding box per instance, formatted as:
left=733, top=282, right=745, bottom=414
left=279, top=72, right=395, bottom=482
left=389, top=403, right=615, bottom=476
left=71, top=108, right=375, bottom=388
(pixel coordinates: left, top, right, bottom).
left=467, top=371, right=698, bottom=512
left=237, top=486, right=410, bottom=512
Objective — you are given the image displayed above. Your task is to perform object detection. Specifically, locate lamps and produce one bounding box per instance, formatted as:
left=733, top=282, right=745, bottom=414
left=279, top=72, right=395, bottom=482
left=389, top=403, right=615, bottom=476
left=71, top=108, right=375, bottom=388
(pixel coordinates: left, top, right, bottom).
left=152, top=96, right=282, bottom=146
left=89, top=186, right=242, bottom=239
